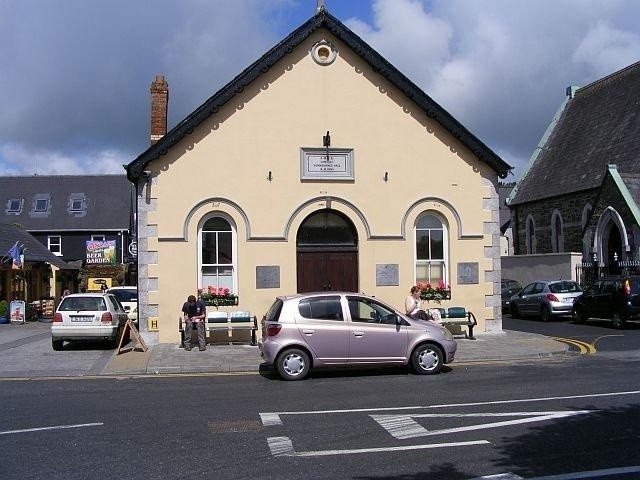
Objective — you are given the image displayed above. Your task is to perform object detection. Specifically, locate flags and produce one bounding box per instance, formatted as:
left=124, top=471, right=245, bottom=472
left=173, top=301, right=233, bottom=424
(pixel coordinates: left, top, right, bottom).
left=3, top=239, right=27, bottom=272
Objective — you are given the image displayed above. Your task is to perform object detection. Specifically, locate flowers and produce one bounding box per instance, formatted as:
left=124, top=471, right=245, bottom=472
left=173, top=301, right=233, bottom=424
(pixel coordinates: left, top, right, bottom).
left=202, top=286, right=230, bottom=306
left=417, top=280, right=448, bottom=301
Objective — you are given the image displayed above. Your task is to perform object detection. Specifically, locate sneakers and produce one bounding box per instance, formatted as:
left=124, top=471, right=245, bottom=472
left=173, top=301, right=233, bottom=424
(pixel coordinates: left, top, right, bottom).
left=185, top=345, right=192, bottom=351
left=199, top=346, right=206, bottom=351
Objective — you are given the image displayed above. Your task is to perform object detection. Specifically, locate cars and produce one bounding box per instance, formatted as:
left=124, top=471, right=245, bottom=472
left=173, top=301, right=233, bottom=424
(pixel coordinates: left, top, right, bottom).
left=572, top=275, right=639, bottom=328
left=509, top=275, right=586, bottom=322
left=101, top=286, right=138, bottom=327
left=259, top=292, right=456, bottom=382
left=50, top=293, right=131, bottom=350
left=501, top=278, right=522, bottom=311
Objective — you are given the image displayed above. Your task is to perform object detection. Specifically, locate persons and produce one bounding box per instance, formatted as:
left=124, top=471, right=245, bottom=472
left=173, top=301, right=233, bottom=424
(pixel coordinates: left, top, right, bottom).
left=405, top=284, right=442, bottom=326
left=100, top=283, right=108, bottom=293
left=60, top=289, right=72, bottom=303
left=181, top=295, right=208, bottom=352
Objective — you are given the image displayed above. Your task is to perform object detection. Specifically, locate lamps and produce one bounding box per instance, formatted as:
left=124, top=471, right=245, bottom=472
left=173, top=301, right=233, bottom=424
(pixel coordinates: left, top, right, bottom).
left=323, top=131, right=330, bottom=161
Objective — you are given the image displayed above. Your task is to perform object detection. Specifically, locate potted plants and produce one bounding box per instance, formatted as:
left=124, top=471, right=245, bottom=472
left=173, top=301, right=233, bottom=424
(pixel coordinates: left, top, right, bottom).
left=25, top=308, right=31, bottom=319
left=0, top=300, right=9, bottom=324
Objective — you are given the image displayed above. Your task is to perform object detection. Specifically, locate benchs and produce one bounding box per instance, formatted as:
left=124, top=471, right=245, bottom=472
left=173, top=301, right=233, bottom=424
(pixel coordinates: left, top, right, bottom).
left=179, top=311, right=258, bottom=348
left=426, top=307, right=477, bottom=340
